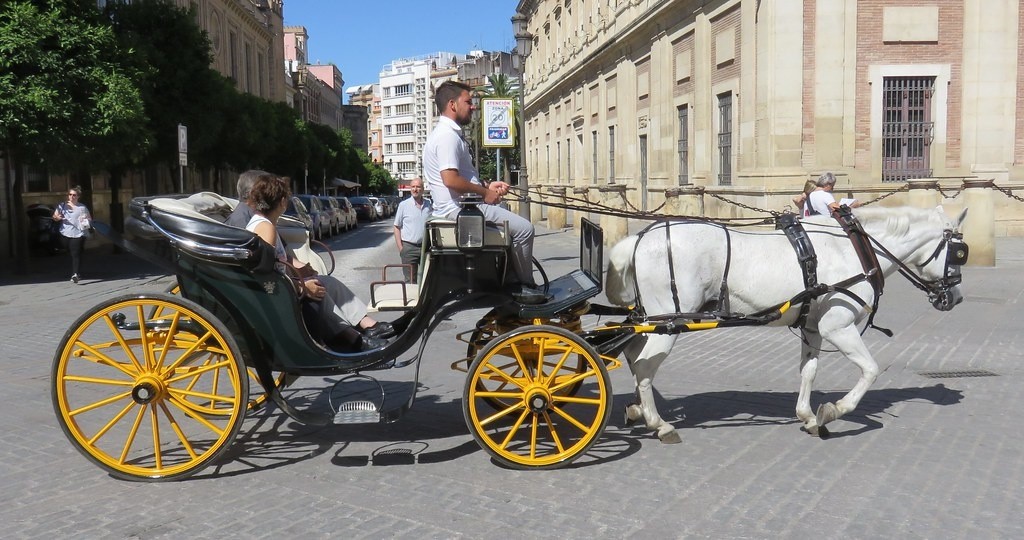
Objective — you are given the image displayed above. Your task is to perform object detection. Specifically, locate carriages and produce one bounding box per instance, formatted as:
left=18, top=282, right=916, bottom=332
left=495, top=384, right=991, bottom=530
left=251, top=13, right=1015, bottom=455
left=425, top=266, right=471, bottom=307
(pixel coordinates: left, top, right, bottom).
left=50, top=192, right=968, bottom=483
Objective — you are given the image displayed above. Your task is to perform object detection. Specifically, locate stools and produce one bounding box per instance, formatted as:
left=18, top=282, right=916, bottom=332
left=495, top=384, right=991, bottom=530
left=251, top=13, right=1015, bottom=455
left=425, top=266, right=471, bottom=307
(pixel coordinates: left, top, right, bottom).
left=431, top=213, right=513, bottom=250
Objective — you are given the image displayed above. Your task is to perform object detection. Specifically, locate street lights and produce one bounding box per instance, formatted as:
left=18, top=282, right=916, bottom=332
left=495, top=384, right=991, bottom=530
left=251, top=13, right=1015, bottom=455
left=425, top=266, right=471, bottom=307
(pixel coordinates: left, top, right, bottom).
left=510, top=10, right=538, bottom=222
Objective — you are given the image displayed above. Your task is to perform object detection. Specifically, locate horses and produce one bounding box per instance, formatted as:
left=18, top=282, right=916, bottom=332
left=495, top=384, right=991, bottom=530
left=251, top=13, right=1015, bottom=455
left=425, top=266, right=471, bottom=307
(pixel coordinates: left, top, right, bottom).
left=604, top=204, right=970, bottom=444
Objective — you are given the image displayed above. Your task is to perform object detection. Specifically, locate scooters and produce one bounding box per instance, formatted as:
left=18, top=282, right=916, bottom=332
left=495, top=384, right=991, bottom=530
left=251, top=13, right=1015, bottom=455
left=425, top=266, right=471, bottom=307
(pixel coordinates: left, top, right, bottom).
left=27, top=204, right=85, bottom=254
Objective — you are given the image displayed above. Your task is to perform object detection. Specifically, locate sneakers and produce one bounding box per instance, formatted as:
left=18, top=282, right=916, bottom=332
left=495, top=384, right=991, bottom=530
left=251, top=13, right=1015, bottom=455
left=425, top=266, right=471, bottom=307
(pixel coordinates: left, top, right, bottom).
left=71, top=274, right=81, bottom=283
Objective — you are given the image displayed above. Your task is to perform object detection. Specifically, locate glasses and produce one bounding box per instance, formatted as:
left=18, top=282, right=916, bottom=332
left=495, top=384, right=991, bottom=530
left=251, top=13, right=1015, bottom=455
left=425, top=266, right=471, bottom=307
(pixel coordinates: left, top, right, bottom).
left=69, top=194, right=76, bottom=197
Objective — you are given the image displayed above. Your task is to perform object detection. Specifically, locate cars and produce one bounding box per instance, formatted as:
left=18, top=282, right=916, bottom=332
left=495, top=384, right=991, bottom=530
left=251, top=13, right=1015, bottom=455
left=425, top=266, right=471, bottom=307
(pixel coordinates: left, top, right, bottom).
left=283, top=197, right=314, bottom=239
left=319, top=196, right=349, bottom=234
left=295, top=194, right=333, bottom=240
left=369, top=197, right=384, bottom=219
left=348, top=197, right=377, bottom=222
left=384, top=196, right=401, bottom=216
left=335, top=197, right=359, bottom=232
left=379, top=198, right=391, bottom=218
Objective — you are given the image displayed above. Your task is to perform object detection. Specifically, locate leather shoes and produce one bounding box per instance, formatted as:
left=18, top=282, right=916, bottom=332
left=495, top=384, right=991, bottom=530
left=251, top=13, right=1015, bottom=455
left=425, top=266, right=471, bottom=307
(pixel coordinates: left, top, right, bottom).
left=508, top=282, right=545, bottom=303
left=352, top=335, right=388, bottom=352
left=360, top=321, right=395, bottom=340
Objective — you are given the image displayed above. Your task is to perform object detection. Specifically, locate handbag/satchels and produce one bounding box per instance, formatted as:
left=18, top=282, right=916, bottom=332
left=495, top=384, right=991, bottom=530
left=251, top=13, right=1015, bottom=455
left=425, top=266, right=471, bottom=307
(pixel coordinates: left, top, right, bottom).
left=84, top=226, right=97, bottom=241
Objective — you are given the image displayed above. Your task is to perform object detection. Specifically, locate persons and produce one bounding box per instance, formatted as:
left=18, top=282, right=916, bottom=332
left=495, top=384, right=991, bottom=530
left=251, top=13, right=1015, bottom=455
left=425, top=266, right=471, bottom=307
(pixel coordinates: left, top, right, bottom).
left=793, top=173, right=860, bottom=218
left=394, top=178, right=451, bottom=320
left=222, top=170, right=398, bottom=351
left=422, top=81, right=555, bottom=303
left=53, top=186, right=92, bottom=283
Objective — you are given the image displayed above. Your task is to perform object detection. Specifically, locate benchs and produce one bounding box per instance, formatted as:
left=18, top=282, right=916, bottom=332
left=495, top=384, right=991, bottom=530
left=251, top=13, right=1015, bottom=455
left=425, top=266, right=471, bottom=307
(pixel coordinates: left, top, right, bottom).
left=168, top=227, right=387, bottom=376
